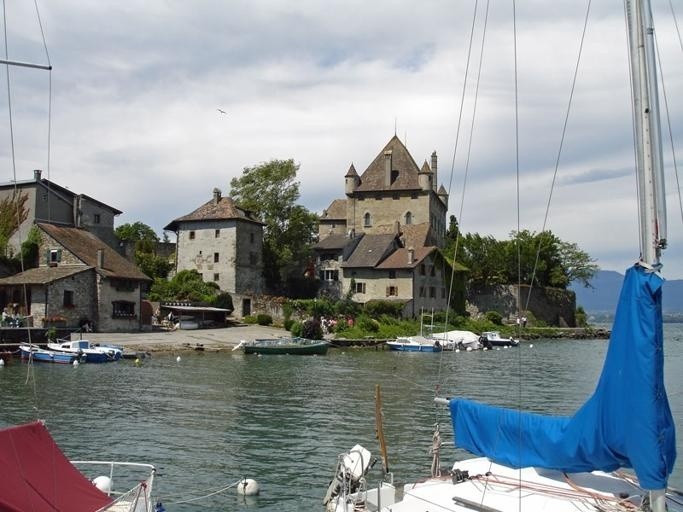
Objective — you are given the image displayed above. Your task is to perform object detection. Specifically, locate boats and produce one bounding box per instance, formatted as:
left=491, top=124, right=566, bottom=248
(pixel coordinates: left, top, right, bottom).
left=384, top=330, right=519, bottom=351
left=231, top=339, right=328, bottom=357
left=0, top=339, right=151, bottom=364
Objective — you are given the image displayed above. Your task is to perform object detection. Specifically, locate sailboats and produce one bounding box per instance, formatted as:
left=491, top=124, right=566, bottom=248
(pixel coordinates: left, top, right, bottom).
left=0, top=0, right=162, bottom=512
left=322, top=0, right=682, bottom=512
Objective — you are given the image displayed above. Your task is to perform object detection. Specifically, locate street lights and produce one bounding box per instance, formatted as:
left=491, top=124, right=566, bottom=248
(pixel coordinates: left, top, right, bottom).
left=312, top=297, right=317, bottom=318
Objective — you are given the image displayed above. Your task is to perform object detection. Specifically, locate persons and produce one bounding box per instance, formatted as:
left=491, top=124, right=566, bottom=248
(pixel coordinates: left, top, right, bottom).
left=1, top=307, right=12, bottom=324
left=319, top=315, right=353, bottom=334
left=150, top=305, right=176, bottom=328
left=516, top=316, right=520, bottom=326
left=521, top=314, right=527, bottom=328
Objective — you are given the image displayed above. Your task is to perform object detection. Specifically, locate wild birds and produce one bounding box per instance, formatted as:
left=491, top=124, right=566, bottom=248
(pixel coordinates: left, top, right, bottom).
left=217, top=108, right=226, bottom=115
